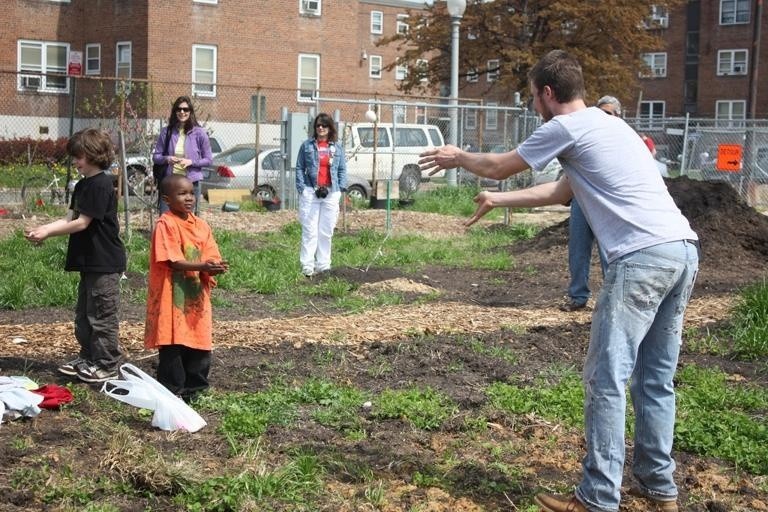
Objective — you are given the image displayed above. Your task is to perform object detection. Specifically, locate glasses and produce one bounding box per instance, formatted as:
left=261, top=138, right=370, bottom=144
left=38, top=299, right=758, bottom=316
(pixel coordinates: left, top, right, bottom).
left=176, top=107, right=190, bottom=113
left=316, top=123, right=329, bottom=128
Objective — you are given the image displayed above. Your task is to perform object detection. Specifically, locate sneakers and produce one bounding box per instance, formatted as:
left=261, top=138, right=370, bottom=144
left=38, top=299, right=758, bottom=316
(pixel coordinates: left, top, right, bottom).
left=532, top=492, right=589, bottom=511
left=58, top=358, right=96, bottom=376
left=562, top=301, right=586, bottom=311
left=77, top=364, right=119, bottom=383
left=621, top=486, right=679, bottom=512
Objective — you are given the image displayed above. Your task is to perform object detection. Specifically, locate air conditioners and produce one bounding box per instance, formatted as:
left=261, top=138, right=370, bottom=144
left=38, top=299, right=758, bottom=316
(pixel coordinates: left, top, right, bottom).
left=307, top=0, right=320, bottom=13
left=733, top=65, right=745, bottom=74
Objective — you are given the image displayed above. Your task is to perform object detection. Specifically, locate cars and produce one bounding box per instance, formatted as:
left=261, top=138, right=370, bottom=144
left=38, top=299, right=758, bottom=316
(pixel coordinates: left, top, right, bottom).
left=702, top=146, right=768, bottom=191
left=193, top=139, right=373, bottom=205
left=461, top=141, right=516, bottom=185
left=535, top=156, right=565, bottom=183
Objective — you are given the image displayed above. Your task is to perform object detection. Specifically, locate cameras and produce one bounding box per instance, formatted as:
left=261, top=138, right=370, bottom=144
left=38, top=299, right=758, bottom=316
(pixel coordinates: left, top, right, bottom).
left=314, top=186, right=329, bottom=200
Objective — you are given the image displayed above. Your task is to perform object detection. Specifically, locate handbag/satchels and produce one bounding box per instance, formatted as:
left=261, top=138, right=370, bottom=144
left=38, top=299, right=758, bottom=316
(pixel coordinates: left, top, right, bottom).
left=153, top=152, right=169, bottom=181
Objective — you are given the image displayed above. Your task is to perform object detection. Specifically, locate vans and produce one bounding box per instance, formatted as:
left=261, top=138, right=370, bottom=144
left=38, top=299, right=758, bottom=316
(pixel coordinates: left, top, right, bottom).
left=318, top=116, right=451, bottom=192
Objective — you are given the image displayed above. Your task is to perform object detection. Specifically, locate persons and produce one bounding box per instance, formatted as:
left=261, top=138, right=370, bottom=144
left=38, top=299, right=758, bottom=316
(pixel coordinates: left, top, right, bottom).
left=417, top=47, right=701, bottom=512
left=641, top=133, right=657, bottom=157
left=560, top=95, right=623, bottom=313
left=145, top=172, right=231, bottom=401
left=153, top=94, right=213, bottom=216
left=22, top=126, right=128, bottom=382
left=296, top=113, right=347, bottom=278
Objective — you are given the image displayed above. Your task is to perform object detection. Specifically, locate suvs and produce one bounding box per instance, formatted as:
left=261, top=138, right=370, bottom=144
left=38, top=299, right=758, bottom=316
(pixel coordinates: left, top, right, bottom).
left=89, top=122, right=225, bottom=197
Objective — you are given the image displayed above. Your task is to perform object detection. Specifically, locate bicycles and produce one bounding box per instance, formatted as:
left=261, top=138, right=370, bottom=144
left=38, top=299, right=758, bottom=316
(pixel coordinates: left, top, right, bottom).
left=23, top=168, right=93, bottom=201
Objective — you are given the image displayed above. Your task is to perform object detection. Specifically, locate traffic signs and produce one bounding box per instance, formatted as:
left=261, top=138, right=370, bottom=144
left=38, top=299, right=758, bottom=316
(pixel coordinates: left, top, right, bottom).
left=717, top=146, right=742, bottom=171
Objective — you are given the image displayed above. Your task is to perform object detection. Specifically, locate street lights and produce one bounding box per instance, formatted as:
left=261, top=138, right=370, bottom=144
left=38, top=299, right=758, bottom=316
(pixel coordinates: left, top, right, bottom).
left=439, top=0, right=465, bottom=189
